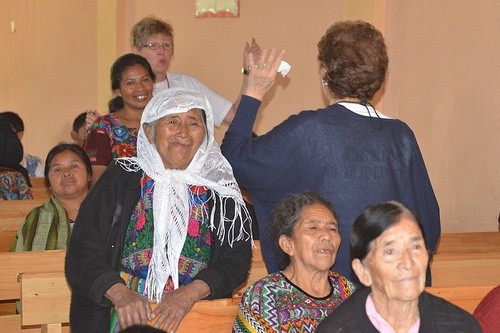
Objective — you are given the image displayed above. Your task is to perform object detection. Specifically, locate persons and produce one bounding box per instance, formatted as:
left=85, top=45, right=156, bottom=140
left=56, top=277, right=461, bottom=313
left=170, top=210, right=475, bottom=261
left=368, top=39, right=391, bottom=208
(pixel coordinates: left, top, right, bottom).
left=0, top=14, right=500, bottom=333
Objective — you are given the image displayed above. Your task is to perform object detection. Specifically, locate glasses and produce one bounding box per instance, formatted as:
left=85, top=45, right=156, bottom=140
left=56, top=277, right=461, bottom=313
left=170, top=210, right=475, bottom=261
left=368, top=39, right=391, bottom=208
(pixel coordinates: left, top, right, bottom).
left=143, top=41, right=173, bottom=50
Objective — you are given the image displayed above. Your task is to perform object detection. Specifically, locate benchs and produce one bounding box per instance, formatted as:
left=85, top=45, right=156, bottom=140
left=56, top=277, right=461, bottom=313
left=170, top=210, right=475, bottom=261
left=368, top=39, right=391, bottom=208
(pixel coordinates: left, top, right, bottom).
left=0, top=165, right=500, bottom=333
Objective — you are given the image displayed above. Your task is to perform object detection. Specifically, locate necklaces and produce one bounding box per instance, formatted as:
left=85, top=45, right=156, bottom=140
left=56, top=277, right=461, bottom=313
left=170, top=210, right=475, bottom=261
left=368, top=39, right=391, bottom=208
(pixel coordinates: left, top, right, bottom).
left=146, top=75, right=171, bottom=100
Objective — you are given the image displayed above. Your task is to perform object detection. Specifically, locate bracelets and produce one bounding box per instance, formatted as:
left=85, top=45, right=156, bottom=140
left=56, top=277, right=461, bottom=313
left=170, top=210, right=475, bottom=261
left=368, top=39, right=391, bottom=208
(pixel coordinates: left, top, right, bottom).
left=241, top=68, right=249, bottom=77
